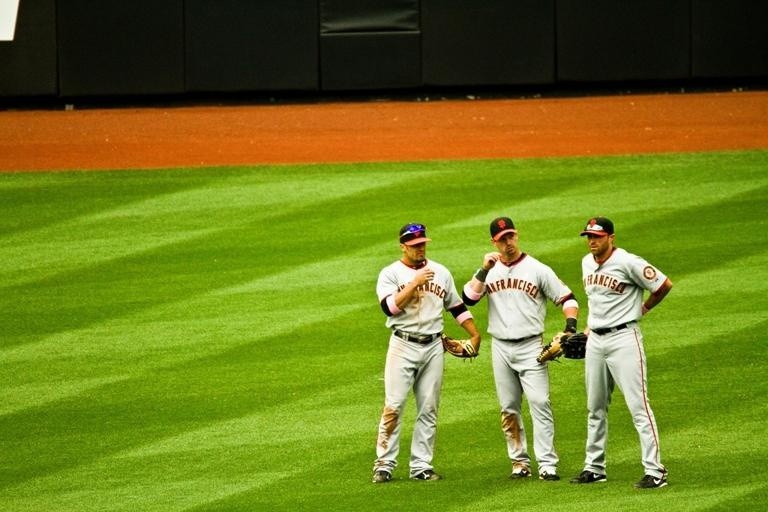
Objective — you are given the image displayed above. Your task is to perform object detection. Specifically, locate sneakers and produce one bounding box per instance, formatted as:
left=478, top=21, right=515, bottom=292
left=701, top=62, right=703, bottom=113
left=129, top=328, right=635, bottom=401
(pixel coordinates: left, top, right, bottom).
left=633, top=475, right=668, bottom=489
left=371, top=471, right=391, bottom=483
left=413, top=470, right=442, bottom=481
left=509, top=469, right=532, bottom=479
left=538, top=471, right=560, bottom=480
left=570, top=470, right=607, bottom=484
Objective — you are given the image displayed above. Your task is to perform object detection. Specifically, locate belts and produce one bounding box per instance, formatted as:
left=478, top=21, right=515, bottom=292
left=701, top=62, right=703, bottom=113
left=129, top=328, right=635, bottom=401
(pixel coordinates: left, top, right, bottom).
left=590, top=325, right=627, bottom=335
left=394, top=329, right=440, bottom=343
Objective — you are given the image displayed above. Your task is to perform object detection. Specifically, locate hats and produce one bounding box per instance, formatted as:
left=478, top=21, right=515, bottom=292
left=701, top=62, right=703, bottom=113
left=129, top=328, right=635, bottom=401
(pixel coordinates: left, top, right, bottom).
left=399, top=223, right=433, bottom=247
left=579, top=215, right=614, bottom=237
left=490, top=218, right=518, bottom=241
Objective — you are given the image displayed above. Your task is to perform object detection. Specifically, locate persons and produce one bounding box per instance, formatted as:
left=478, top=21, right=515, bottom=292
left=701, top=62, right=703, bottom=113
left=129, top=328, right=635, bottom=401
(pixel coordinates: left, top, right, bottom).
left=462, top=217, right=581, bottom=482
left=567, top=216, right=674, bottom=489
left=372, top=223, right=481, bottom=484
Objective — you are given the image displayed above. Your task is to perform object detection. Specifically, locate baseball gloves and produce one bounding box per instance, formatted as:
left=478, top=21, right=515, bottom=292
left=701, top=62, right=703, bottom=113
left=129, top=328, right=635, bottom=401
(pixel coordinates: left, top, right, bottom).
left=442, top=332, right=478, bottom=358
left=536, top=332, right=573, bottom=363
left=560, top=332, right=587, bottom=358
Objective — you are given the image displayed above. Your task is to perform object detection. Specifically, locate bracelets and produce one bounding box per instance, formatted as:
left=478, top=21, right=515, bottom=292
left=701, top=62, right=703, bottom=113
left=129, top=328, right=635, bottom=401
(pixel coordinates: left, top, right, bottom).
left=642, top=302, right=649, bottom=316
left=475, top=267, right=488, bottom=283
left=564, top=317, right=577, bottom=333
